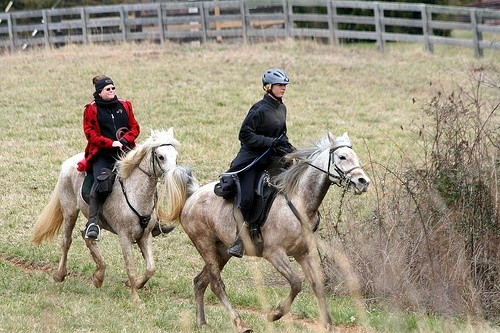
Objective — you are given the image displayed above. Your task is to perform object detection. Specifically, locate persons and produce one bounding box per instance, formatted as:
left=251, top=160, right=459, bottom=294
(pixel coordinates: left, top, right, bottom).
left=227, top=69, right=302, bottom=258
left=81, top=74, right=175, bottom=239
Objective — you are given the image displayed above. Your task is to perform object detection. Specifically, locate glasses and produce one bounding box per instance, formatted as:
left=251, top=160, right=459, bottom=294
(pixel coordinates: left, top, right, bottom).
left=102, top=87, right=115, bottom=91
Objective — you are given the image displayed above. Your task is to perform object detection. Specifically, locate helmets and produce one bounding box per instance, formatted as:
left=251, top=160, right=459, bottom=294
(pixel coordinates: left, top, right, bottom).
left=262, top=70, right=290, bottom=86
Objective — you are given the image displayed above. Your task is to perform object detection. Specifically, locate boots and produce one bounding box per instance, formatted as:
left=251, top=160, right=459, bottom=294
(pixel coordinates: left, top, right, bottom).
left=230, top=222, right=254, bottom=258
left=151, top=217, right=175, bottom=238
left=86, top=193, right=107, bottom=237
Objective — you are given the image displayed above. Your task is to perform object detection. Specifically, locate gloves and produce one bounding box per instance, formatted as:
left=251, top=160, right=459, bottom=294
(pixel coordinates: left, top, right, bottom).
left=272, top=139, right=288, bottom=149
left=119, top=138, right=129, bottom=149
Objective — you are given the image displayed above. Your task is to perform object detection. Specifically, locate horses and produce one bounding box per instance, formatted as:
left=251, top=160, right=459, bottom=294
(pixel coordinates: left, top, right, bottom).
left=179, top=128, right=371, bottom=333
left=31, top=126, right=187, bottom=311
